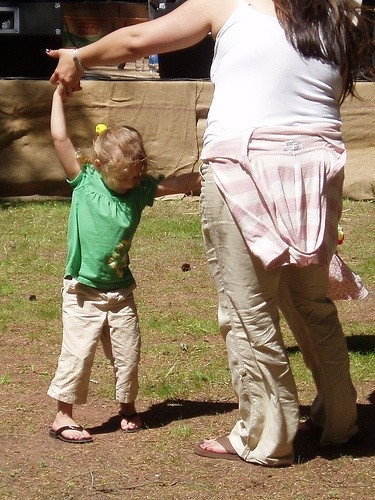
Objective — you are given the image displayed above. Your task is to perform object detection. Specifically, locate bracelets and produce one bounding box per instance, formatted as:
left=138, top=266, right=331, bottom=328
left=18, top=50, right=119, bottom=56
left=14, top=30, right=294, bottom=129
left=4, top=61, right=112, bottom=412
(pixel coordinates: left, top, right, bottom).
left=73, top=49, right=88, bottom=71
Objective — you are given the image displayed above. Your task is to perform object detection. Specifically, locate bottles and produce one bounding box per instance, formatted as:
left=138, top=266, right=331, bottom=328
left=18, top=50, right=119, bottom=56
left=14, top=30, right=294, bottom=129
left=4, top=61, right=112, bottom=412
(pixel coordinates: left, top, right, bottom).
left=149, top=54, right=159, bottom=77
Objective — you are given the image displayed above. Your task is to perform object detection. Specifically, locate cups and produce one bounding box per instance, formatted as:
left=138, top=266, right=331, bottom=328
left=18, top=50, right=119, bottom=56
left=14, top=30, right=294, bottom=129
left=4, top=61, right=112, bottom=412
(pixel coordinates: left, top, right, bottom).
left=135, top=57, right=144, bottom=72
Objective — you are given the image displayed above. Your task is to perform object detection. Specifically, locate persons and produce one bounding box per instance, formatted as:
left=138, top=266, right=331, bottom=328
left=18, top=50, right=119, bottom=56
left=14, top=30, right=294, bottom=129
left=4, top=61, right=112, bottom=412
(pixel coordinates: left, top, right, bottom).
left=47, top=84, right=204, bottom=444
left=46, top=0, right=375, bottom=466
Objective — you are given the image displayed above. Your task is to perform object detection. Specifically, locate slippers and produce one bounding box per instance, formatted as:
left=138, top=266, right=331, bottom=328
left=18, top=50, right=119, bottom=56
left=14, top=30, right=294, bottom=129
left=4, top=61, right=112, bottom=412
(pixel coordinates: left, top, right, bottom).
left=118, top=409, right=142, bottom=433
left=48, top=425, right=93, bottom=443
left=298, top=418, right=360, bottom=443
left=193, top=434, right=242, bottom=460
